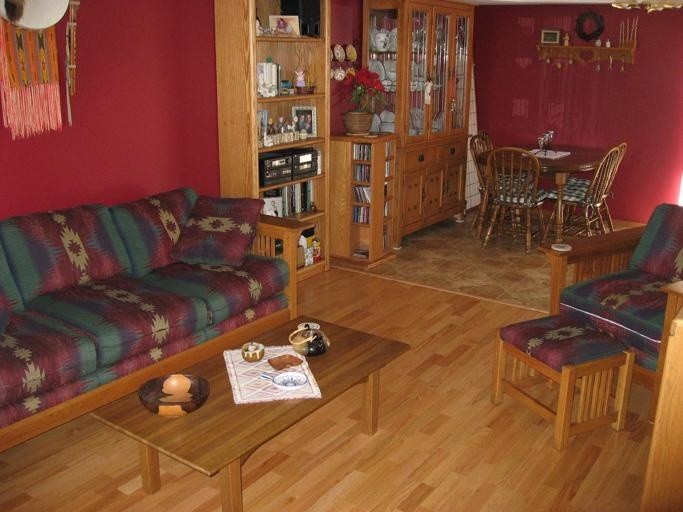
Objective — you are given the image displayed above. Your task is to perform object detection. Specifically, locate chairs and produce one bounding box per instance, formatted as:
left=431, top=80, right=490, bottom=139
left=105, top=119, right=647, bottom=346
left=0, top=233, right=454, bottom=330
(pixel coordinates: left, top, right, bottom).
left=470, top=133, right=627, bottom=256
left=536, top=204, right=683, bottom=426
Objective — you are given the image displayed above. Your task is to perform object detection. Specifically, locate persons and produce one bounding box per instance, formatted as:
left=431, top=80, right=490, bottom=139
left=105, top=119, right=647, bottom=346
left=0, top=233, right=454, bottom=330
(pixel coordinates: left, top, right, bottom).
left=298, top=113, right=304, bottom=130
left=274, top=17, right=293, bottom=33
left=310, top=238, right=320, bottom=262
left=305, top=113, right=311, bottom=133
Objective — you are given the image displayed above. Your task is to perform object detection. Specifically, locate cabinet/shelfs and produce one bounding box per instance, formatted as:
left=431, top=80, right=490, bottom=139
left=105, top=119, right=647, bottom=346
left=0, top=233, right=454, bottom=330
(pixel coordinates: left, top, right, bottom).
left=213, top=1, right=331, bottom=285
left=329, top=132, right=397, bottom=270
left=362, top=1, right=476, bottom=251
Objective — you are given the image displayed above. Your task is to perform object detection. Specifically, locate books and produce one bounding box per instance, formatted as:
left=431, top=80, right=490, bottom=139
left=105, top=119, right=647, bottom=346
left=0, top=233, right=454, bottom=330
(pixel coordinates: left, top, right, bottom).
left=256, top=61, right=293, bottom=99
left=257, top=109, right=269, bottom=150
left=520, top=148, right=571, bottom=160
left=352, top=142, right=392, bottom=258
left=259, top=179, right=318, bottom=219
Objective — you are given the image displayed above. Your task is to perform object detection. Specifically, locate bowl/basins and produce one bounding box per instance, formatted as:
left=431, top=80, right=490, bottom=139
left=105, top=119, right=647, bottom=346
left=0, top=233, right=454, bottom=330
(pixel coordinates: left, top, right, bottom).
left=137, top=373, right=210, bottom=418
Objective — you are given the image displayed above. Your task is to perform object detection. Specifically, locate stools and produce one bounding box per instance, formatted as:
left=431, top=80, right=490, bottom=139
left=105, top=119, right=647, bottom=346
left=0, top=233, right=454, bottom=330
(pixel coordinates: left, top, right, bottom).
left=490, top=313, right=636, bottom=452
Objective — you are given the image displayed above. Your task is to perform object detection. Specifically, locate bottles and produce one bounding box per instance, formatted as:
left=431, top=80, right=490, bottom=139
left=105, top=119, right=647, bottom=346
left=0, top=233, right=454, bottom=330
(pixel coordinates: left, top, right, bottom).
left=264, top=117, right=299, bottom=145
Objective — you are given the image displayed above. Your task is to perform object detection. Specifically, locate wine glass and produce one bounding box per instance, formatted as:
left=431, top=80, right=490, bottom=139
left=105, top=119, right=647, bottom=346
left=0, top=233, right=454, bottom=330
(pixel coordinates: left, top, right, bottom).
left=537, top=128, right=556, bottom=157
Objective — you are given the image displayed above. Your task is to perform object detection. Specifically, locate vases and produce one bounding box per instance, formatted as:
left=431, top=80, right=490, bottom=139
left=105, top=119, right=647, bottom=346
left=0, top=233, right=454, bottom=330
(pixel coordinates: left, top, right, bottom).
left=342, top=112, right=372, bottom=136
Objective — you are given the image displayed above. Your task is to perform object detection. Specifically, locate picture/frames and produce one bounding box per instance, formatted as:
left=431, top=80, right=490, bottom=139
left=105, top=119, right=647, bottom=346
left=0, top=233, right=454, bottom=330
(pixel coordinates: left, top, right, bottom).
left=540, top=30, right=560, bottom=44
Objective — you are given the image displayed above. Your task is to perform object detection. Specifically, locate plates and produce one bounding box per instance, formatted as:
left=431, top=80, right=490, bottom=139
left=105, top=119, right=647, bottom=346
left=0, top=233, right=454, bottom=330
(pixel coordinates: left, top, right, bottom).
left=267, top=355, right=304, bottom=370
left=329, top=42, right=358, bottom=82
left=271, top=371, right=307, bottom=390
left=367, top=26, right=442, bottom=91
left=371, top=105, right=446, bottom=135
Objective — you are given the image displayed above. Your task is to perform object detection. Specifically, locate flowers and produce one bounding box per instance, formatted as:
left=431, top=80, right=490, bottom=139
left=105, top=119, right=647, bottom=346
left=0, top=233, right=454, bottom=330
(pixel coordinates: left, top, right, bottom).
left=343, top=67, right=389, bottom=112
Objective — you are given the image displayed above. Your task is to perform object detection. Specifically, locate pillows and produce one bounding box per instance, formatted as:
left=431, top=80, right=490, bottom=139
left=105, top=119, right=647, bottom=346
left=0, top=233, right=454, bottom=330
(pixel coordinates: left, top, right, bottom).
left=0, top=246, right=24, bottom=340
left=109, top=186, right=199, bottom=279
left=168, top=194, right=265, bottom=267
left=1, top=202, right=133, bottom=309
left=627, top=204, right=682, bottom=281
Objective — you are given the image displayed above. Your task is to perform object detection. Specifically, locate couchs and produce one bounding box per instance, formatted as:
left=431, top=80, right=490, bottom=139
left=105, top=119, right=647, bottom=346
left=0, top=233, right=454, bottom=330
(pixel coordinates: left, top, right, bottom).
left=0, top=186, right=315, bottom=453
